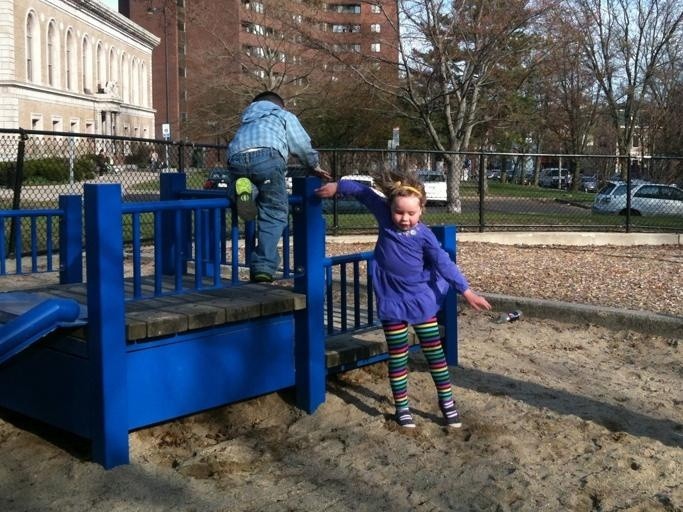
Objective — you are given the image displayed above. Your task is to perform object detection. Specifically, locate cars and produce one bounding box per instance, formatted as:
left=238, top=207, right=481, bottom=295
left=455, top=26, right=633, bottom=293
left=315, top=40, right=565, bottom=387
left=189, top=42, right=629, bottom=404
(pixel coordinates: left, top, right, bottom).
left=415, top=170, right=449, bottom=203
left=283, top=166, right=308, bottom=195
left=207, top=166, right=231, bottom=189
left=341, top=176, right=375, bottom=188
left=473, top=168, right=597, bottom=193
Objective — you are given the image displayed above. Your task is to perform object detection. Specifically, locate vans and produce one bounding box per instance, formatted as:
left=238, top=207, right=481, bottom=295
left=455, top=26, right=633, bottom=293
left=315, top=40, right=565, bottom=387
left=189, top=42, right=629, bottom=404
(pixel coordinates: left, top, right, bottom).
left=592, top=179, right=683, bottom=216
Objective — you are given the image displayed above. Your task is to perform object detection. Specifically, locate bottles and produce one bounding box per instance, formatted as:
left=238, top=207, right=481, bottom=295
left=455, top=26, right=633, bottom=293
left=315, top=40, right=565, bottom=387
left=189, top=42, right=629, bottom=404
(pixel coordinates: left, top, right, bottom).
left=498, top=310, right=523, bottom=323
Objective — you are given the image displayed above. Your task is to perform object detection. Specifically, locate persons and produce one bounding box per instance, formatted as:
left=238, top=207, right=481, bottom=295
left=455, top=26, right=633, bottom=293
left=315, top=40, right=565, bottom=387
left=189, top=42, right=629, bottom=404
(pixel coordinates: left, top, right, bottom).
left=492, top=171, right=497, bottom=177
left=150, top=148, right=157, bottom=173
left=313, top=174, right=493, bottom=429
left=564, top=171, right=572, bottom=190
left=96, top=148, right=108, bottom=181
left=226, top=91, right=334, bottom=283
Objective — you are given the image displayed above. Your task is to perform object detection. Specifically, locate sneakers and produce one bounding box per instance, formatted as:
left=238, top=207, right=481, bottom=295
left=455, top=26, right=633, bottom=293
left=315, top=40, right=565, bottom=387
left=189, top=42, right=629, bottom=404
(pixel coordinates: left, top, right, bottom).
left=235, top=177, right=258, bottom=223
left=438, top=399, right=462, bottom=428
left=394, top=409, right=416, bottom=428
left=254, top=272, right=273, bottom=282
